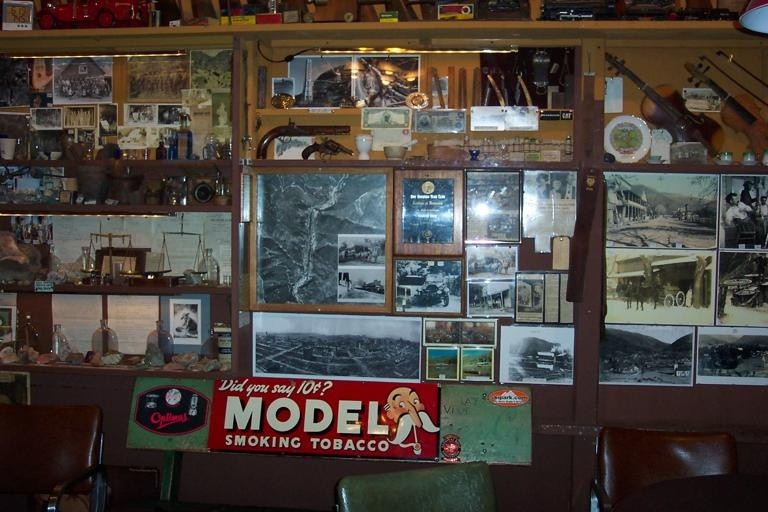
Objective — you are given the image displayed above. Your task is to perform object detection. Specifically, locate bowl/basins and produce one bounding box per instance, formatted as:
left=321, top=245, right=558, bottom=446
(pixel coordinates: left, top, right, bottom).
left=383, top=145, right=408, bottom=160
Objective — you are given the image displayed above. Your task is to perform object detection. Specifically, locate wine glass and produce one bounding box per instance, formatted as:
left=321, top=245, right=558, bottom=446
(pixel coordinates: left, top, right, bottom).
left=355, top=135, right=374, bottom=161
left=469, top=148, right=480, bottom=161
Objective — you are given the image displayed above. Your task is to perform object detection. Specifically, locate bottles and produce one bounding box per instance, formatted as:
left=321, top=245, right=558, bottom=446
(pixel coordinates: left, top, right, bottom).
left=76, top=247, right=95, bottom=268
left=201, top=328, right=219, bottom=360
left=155, top=113, right=232, bottom=160
left=198, top=248, right=220, bottom=285
left=146, top=320, right=174, bottom=359
left=52, top=324, right=66, bottom=360
left=91, top=320, right=118, bottom=355
left=481, top=135, right=572, bottom=155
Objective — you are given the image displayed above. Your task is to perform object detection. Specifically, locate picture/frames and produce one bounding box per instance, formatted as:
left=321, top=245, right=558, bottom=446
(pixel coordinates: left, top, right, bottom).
left=249, top=309, right=423, bottom=387
left=422, top=312, right=498, bottom=385
left=157, top=293, right=212, bottom=355
left=239, top=163, right=578, bottom=327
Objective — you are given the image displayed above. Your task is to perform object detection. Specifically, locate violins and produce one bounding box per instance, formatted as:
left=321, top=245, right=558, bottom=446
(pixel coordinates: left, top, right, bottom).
left=685, top=61, right=767, bottom=160
left=605, top=53, right=725, bottom=162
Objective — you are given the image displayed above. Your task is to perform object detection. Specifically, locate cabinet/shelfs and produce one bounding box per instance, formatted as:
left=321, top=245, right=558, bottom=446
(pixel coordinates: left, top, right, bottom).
left=0, top=20, right=242, bottom=379
left=243, top=37, right=583, bottom=162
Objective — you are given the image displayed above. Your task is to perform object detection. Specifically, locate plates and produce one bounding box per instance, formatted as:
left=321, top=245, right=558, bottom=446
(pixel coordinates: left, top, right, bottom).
left=712, top=160, right=760, bottom=166
left=604, top=115, right=665, bottom=165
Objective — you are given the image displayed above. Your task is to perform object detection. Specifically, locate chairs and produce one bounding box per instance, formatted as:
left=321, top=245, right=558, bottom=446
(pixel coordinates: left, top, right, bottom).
left=1, top=405, right=110, bottom=510
left=334, top=460, right=497, bottom=512
left=589, top=426, right=738, bottom=512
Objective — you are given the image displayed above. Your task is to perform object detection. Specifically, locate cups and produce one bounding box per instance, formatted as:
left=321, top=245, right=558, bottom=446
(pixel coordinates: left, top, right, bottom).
left=720, top=151, right=733, bottom=160
left=223, top=276, right=233, bottom=287
left=743, top=152, right=756, bottom=161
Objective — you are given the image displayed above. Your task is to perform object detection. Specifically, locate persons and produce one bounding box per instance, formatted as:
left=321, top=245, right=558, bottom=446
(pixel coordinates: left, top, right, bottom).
left=752, top=194, right=768, bottom=226
left=179, top=310, right=198, bottom=337
left=740, top=180, right=759, bottom=206
left=545, top=177, right=563, bottom=199
left=536, top=173, right=551, bottom=199
left=634, top=279, right=644, bottom=310
left=561, top=173, right=575, bottom=199
left=623, top=280, right=632, bottom=309
left=724, top=192, right=752, bottom=228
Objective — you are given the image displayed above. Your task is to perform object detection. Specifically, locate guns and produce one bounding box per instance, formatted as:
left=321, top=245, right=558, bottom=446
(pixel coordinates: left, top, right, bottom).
left=256, top=117, right=350, bottom=160
left=301, top=138, right=354, bottom=160
